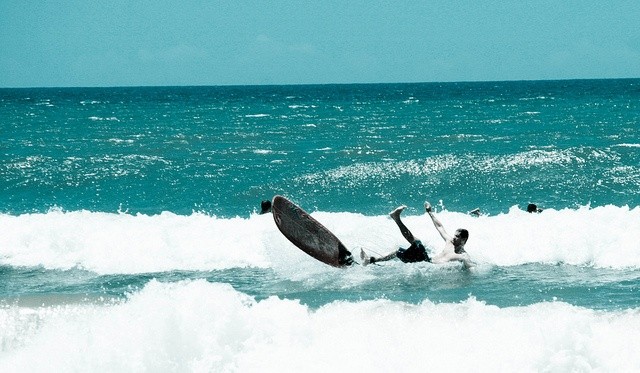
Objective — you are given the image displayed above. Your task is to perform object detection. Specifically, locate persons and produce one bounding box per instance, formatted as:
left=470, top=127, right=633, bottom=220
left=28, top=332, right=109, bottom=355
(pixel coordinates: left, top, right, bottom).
left=361, top=201, right=472, bottom=269
left=259, top=199, right=270, bottom=215
left=471, top=204, right=542, bottom=217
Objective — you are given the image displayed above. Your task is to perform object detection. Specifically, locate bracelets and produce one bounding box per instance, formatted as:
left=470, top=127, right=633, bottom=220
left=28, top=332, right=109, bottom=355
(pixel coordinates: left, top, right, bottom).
left=426, top=207, right=431, bottom=212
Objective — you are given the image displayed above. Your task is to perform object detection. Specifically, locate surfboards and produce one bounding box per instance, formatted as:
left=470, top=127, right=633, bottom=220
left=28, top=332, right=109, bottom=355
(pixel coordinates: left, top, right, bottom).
left=271, top=194, right=353, bottom=266
